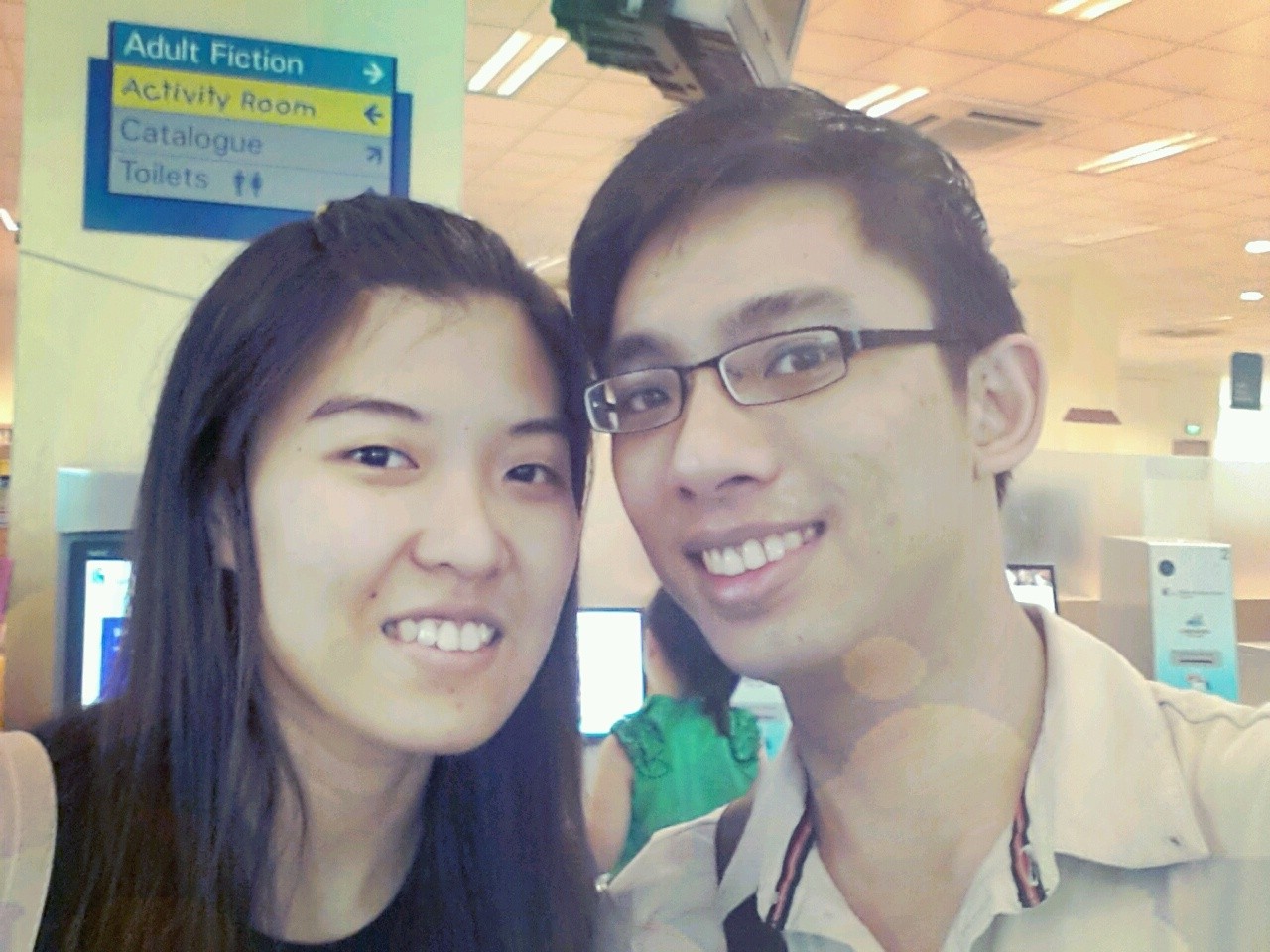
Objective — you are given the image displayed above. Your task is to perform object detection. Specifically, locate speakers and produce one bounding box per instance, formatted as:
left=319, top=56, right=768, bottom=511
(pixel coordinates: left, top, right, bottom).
left=1229, top=352, right=1263, bottom=410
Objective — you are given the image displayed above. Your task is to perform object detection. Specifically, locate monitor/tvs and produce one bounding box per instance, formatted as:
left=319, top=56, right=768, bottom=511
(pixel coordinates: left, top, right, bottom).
left=622, top=0, right=811, bottom=90
left=575, top=606, right=648, bottom=737
left=1004, top=564, right=1058, bottom=616
left=62, top=537, right=137, bottom=714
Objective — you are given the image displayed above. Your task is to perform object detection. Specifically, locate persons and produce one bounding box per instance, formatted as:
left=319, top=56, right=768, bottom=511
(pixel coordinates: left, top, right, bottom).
left=1, top=188, right=595, bottom=951
left=581, top=584, right=770, bottom=885
left=567, top=88, right=1269, bottom=952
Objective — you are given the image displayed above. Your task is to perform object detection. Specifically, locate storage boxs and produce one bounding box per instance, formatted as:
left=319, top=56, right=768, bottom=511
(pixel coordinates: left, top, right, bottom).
left=1102, top=532, right=1237, bottom=708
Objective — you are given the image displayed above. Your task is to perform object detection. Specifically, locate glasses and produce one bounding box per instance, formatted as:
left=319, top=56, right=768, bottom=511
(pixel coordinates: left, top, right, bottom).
left=582, top=324, right=959, bottom=439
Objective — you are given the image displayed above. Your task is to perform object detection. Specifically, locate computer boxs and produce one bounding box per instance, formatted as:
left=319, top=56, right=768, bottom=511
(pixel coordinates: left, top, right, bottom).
left=1098, top=534, right=1242, bottom=701
